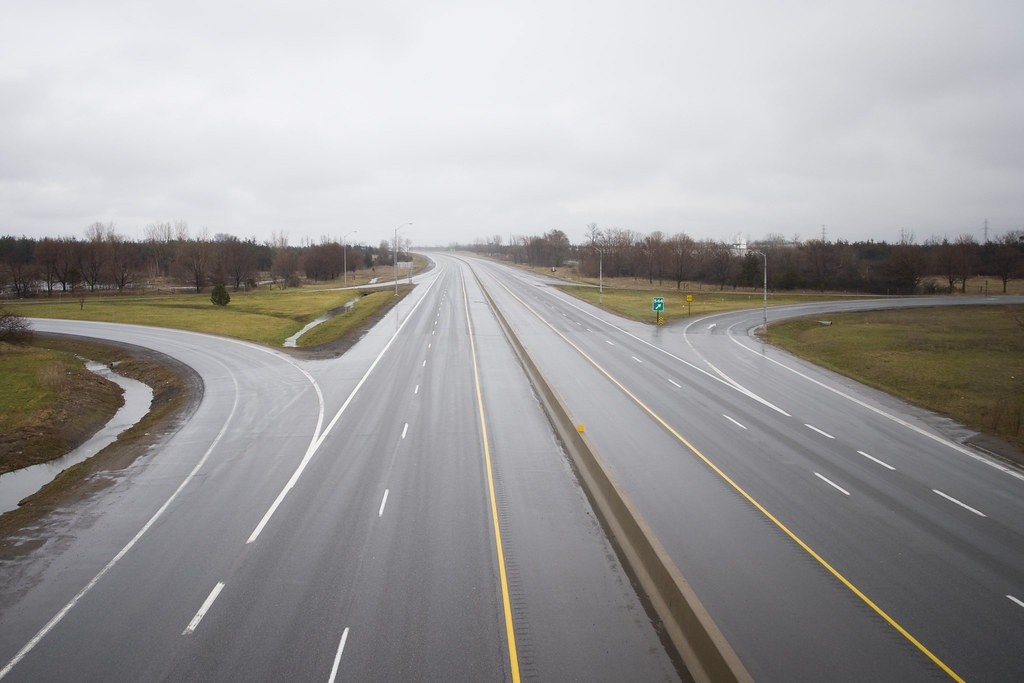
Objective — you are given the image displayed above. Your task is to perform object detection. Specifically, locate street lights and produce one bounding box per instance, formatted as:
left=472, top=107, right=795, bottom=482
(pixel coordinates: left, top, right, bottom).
left=343, top=229, right=359, bottom=288
left=591, top=245, right=602, bottom=304
left=756, top=250, right=768, bottom=333
left=394, top=221, right=414, bottom=298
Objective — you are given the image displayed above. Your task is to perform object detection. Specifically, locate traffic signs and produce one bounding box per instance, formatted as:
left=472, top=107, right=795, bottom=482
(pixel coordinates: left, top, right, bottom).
left=652, top=297, right=664, bottom=311
